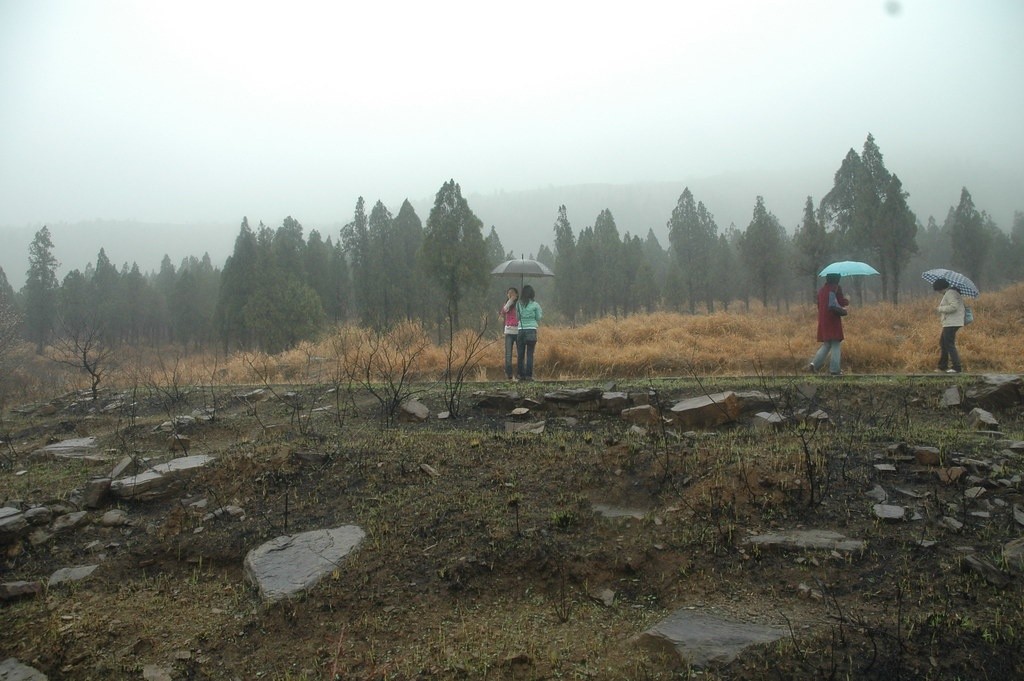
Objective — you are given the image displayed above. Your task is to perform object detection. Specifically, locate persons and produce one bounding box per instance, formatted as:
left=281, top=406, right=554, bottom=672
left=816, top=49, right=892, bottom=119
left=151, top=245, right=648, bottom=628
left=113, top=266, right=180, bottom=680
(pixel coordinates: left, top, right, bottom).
left=502, top=287, right=519, bottom=380
left=932, top=279, right=964, bottom=373
left=810, top=273, right=848, bottom=376
left=516, top=285, right=542, bottom=379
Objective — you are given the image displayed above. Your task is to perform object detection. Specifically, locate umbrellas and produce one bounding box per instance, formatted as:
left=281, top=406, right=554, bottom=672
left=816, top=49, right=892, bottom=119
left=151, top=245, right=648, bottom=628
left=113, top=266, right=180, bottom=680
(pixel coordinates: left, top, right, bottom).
left=818, top=261, right=881, bottom=293
left=921, top=269, right=980, bottom=298
left=490, top=253, right=556, bottom=290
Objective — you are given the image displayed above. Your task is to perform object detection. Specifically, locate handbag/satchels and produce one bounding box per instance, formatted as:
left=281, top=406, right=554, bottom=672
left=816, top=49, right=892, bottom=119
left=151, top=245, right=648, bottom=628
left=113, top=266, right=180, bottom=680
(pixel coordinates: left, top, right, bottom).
left=828, top=284, right=847, bottom=315
left=964, top=307, right=973, bottom=324
left=524, top=329, right=538, bottom=344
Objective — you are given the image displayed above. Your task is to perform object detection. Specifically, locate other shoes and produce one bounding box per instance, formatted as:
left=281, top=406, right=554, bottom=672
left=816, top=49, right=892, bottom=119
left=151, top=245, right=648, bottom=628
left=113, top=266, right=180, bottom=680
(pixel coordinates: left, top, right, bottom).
left=810, top=363, right=819, bottom=373
left=934, top=368, right=943, bottom=372
left=947, top=369, right=958, bottom=373
